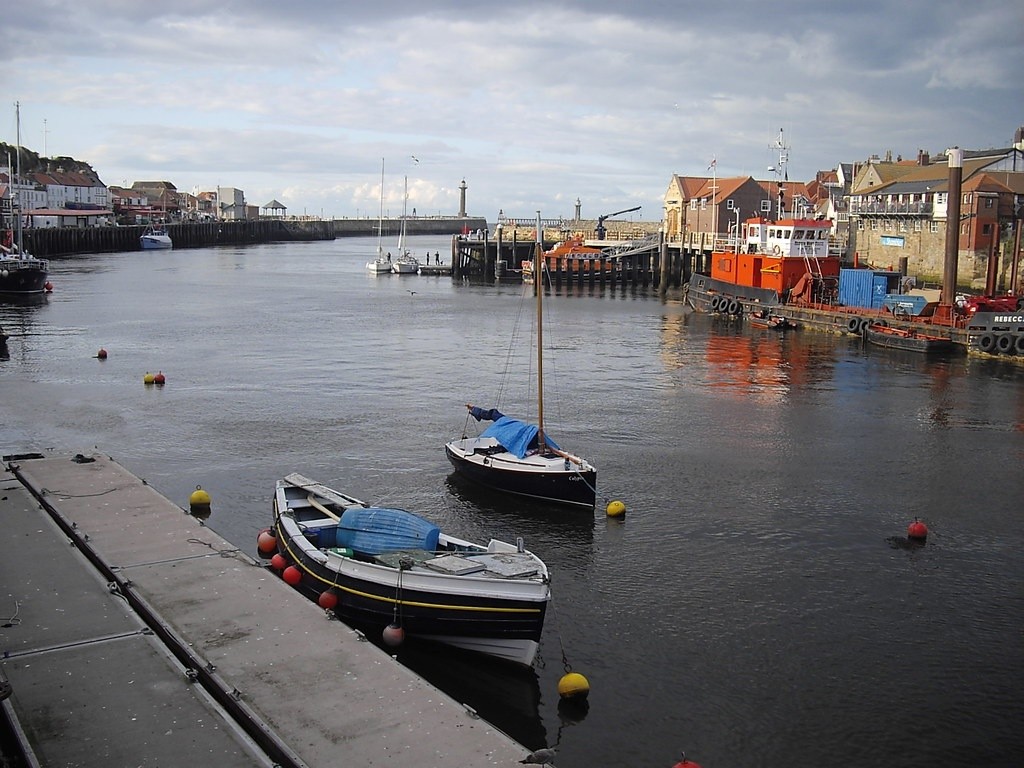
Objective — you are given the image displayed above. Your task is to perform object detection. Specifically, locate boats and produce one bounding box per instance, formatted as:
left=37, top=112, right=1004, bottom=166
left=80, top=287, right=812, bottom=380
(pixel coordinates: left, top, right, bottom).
left=864, top=322, right=952, bottom=356
left=745, top=311, right=786, bottom=331
left=272, top=472, right=555, bottom=669
left=140, top=220, right=173, bottom=249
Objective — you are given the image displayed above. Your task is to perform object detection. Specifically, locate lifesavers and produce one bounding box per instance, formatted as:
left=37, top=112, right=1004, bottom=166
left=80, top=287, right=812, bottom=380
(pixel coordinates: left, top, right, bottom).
left=847, top=316, right=888, bottom=334
left=976, top=331, right=1024, bottom=355
left=710, top=294, right=740, bottom=314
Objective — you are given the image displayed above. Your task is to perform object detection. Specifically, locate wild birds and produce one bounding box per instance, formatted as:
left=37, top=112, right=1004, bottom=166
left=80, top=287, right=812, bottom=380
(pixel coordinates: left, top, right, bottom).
left=411, top=155, right=419, bottom=162
left=406, top=289, right=418, bottom=296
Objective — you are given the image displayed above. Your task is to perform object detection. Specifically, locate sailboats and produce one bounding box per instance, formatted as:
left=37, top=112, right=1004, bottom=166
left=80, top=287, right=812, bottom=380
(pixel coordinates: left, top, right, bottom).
left=444, top=209, right=600, bottom=513
left=366, top=157, right=451, bottom=278
left=1, top=100, right=55, bottom=294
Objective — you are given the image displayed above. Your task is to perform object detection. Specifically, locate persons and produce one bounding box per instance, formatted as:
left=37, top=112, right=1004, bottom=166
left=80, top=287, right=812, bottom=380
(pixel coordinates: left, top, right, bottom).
left=476, top=227, right=482, bottom=241
left=435, top=251, right=439, bottom=265
left=385, top=252, right=391, bottom=263
left=426, top=252, right=429, bottom=265
